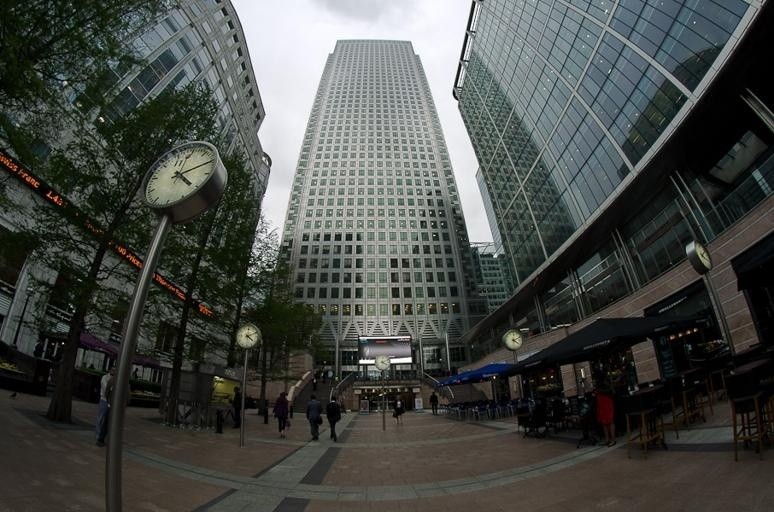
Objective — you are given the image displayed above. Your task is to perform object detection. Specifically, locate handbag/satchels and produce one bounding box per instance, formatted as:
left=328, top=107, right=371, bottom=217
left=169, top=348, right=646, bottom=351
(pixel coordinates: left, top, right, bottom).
left=316, top=416, right=322, bottom=424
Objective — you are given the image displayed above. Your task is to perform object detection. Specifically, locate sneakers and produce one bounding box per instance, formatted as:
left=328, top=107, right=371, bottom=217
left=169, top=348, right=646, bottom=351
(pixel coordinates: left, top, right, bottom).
left=95, top=440, right=104, bottom=447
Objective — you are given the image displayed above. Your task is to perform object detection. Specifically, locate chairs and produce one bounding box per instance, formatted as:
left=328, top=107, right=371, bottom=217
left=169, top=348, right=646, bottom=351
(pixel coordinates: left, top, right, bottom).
left=437, top=336, right=773, bottom=460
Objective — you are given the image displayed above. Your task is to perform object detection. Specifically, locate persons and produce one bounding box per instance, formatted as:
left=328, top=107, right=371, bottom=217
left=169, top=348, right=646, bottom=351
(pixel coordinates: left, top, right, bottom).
left=105, top=370, right=131, bottom=407
left=326, top=396, right=341, bottom=442
left=229, top=386, right=242, bottom=429
left=132, top=367, right=139, bottom=379
left=312, top=377, right=318, bottom=391
left=429, top=391, right=439, bottom=416
left=306, top=394, right=321, bottom=440
left=33, top=341, right=45, bottom=358
left=393, top=394, right=404, bottom=425
left=594, top=371, right=617, bottom=447
left=96, top=365, right=116, bottom=447
left=367, top=391, right=373, bottom=413
left=272, top=393, right=288, bottom=439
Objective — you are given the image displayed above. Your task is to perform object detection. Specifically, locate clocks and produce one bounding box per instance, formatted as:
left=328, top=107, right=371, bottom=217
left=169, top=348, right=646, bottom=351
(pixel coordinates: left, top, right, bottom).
left=503, top=329, right=527, bottom=430
left=232, top=321, right=265, bottom=450
left=91, top=137, right=228, bottom=510
left=685, top=238, right=735, bottom=357
left=371, top=353, right=390, bottom=431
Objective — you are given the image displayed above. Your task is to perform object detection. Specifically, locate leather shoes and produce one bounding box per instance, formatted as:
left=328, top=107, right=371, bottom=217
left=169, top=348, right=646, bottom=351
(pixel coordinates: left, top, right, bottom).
left=608, top=440, right=616, bottom=447
left=599, top=440, right=609, bottom=446
